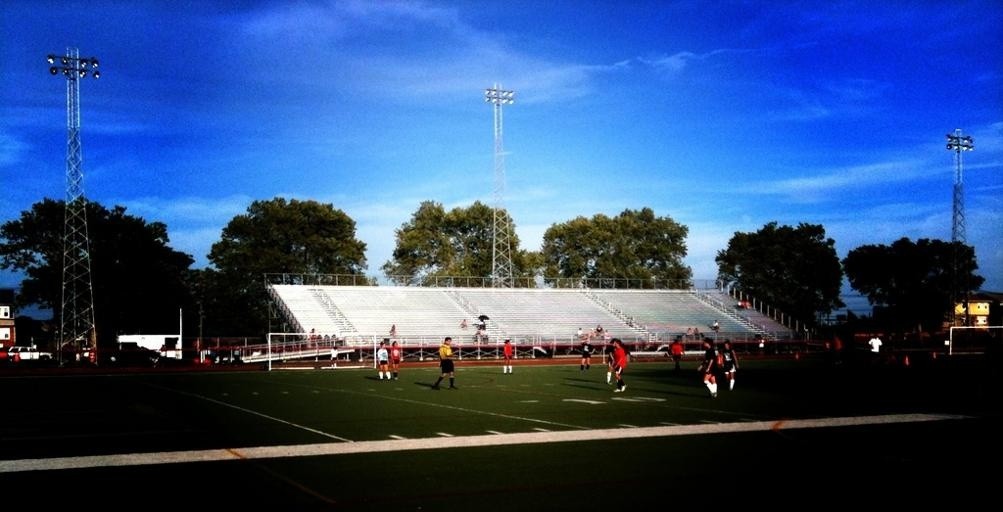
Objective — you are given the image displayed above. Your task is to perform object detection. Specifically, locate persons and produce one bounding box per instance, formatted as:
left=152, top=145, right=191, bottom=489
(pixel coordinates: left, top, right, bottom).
left=686, top=327, right=702, bottom=339
left=310, top=327, right=337, bottom=345
left=868, top=334, right=884, bottom=353
left=712, top=319, right=719, bottom=332
left=606, top=338, right=628, bottom=393
left=330, top=346, right=339, bottom=368
left=604, top=341, right=618, bottom=385
left=670, top=338, right=685, bottom=371
left=390, top=324, right=397, bottom=337
left=697, top=338, right=718, bottom=399
left=758, top=336, right=765, bottom=348
left=721, top=342, right=740, bottom=391
left=503, top=339, right=513, bottom=374
left=376, top=341, right=392, bottom=381
left=577, top=325, right=611, bottom=340
left=460, top=319, right=467, bottom=328
left=737, top=301, right=752, bottom=309
left=474, top=320, right=490, bottom=340
left=432, top=337, right=459, bottom=390
left=580, top=338, right=595, bottom=370
left=390, top=340, right=401, bottom=381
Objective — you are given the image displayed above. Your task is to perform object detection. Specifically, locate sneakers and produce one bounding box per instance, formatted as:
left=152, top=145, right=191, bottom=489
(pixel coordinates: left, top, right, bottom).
left=614, top=384, right=627, bottom=393
left=449, top=385, right=458, bottom=390
left=430, top=385, right=441, bottom=391
